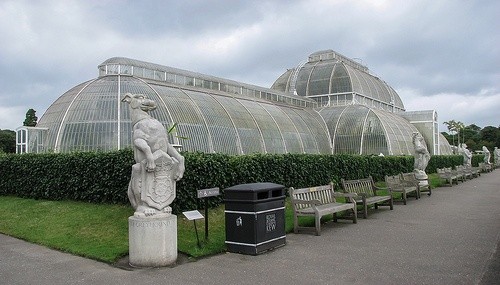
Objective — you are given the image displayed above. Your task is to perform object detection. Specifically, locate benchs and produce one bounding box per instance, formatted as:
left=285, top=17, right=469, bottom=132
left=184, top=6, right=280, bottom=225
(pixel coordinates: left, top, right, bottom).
left=437, top=162, right=491, bottom=187
left=289, top=182, right=357, bottom=235
left=385, top=174, right=418, bottom=205
left=401, top=172, right=431, bottom=198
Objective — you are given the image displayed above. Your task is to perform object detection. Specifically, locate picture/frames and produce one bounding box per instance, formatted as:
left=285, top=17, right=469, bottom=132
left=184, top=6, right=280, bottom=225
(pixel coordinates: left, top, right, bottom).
left=341, top=175, right=392, bottom=218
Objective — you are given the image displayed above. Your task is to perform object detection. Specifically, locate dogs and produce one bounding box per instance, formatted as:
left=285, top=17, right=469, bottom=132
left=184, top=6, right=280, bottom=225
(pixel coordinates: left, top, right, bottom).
left=121, top=92, right=188, bottom=218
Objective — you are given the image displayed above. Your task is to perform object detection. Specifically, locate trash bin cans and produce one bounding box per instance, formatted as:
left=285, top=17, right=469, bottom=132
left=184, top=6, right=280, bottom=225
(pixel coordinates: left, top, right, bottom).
left=222, top=181, right=286, bottom=255
